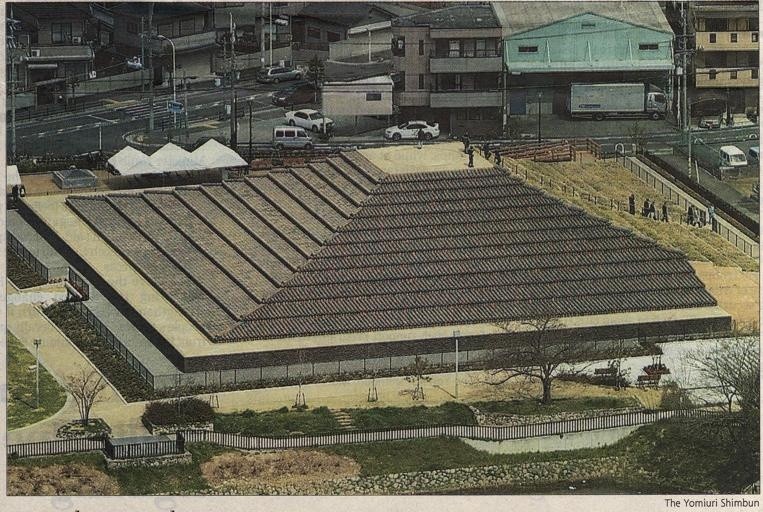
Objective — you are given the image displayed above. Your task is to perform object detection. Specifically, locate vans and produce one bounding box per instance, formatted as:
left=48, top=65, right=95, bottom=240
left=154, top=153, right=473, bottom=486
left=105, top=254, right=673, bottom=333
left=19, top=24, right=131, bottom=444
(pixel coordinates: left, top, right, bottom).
left=273, top=125, right=313, bottom=150
left=718, top=145, right=748, bottom=169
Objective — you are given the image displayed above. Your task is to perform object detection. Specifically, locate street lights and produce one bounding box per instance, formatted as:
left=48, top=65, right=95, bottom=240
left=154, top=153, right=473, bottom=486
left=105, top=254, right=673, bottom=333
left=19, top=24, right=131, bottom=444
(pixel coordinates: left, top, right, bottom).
left=33, top=338, right=42, bottom=408
left=364, top=26, right=373, bottom=62
left=537, top=92, right=542, bottom=143
left=453, top=329, right=461, bottom=401
left=157, top=33, right=177, bottom=128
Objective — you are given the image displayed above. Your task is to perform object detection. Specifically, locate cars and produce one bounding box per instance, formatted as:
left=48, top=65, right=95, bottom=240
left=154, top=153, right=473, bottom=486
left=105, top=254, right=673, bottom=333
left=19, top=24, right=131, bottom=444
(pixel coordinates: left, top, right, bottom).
left=271, top=81, right=321, bottom=107
left=255, top=65, right=302, bottom=83
left=749, top=147, right=759, bottom=164
left=285, top=108, right=334, bottom=133
left=699, top=107, right=724, bottom=129
left=385, top=119, right=441, bottom=140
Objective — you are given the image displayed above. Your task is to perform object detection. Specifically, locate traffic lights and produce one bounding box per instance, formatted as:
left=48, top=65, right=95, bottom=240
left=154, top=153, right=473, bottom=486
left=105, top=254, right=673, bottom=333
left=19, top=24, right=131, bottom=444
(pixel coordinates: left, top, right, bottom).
left=275, top=18, right=288, bottom=26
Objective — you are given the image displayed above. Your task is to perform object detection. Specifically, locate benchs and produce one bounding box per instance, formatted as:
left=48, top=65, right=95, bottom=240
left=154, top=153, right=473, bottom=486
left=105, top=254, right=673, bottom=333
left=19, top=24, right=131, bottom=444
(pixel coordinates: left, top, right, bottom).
left=635, top=376, right=660, bottom=389
left=592, top=368, right=617, bottom=384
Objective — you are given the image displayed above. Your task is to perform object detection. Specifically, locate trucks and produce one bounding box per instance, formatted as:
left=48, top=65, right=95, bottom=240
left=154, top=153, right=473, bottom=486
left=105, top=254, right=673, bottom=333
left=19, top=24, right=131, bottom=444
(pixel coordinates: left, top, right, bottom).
left=564, top=82, right=667, bottom=123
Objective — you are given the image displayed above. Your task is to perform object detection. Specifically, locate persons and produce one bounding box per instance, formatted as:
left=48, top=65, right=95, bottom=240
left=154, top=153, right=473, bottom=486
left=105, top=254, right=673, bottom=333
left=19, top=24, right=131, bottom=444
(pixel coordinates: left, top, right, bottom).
left=629, top=193, right=635, bottom=214
left=495, top=146, right=501, bottom=165
left=650, top=200, right=656, bottom=220
left=694, top=206, right=702, bottom=228
left=662, top=201, right=669, bottom=222
left=644, top=198, right=650, bottom=217
left=483, top=143, right=491, bottom=160
left=417, top=128, right=424, bottom=149
left=461, top=134, right=468, bottom=151
left=467, top=146, right=476, bottom=167
left=708, top=204, right=714, bottom=224
left=466, top=137, right=471, bottom=153
left=688, top=206, right=694, bottom=226
